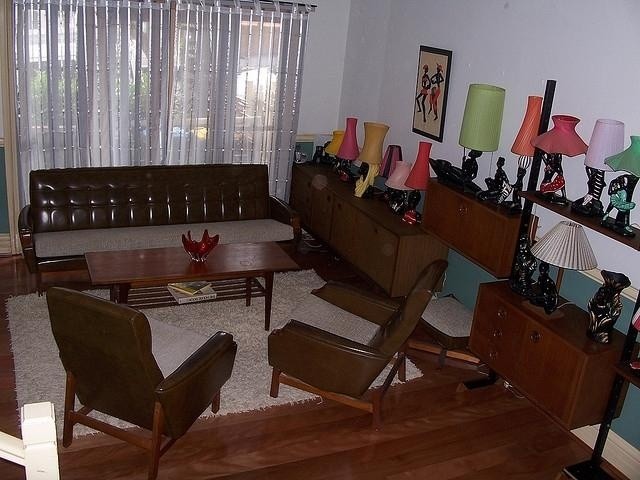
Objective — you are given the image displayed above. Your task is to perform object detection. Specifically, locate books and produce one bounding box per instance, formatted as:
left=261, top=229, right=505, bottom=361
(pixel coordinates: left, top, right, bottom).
left=167, top=280, right=217, bottom=305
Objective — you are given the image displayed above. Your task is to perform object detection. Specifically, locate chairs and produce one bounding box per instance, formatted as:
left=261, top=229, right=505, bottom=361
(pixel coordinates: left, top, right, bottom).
left=43, top=286, right=238, bottom=479
left=265, top=259, right=448, bottom=430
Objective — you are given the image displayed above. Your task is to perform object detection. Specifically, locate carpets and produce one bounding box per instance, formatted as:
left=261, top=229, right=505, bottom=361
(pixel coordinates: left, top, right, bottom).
left=5, top=268, right=425, bottom=439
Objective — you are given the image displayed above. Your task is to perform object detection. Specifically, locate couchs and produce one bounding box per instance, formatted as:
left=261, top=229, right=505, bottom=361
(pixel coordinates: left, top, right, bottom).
left=17, top=164, right=302, bottom=295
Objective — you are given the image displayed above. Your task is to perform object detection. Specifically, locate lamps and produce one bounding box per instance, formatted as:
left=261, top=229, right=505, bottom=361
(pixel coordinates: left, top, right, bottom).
left=584, top=120, right=624, bottom=209
left=530, top=116, right=589, bottom=201
left=357, top=122, right=390, bottom=198
left=379, top=145, right=400, bottom=178
left=386, top=162, right=412, bottom=211
left=325, top=130, right=343, bottom=175
left=336, top=119, right=359, bottom=184
left=458, top=83, right=505, bottom=189
left=510, top=96, right=542, bottom=212
left=604, top=137, right=640, bottom=229
left=530, top=221, right=596, bottom=318
left=406, top=142, right=430, bottom=219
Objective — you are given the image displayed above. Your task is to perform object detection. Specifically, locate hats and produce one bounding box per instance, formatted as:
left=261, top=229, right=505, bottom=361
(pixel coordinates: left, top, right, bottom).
left=436, top=63, right=443, bottom=73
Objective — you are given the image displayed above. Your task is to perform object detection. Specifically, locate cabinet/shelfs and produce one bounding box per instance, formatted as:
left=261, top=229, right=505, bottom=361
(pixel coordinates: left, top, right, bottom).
left=420, top=174, right=538, bottom=278
left=333, top=187, right=450, bottom=300
left=292, top=162, right=334, bottom=247
left=467, top=279, right=636, bottom=433
left=561, top=293, right=639, bottom=480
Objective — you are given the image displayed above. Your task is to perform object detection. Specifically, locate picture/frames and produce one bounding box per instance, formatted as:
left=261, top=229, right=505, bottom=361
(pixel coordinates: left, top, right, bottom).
left=412, top=46, right=453, bottom=143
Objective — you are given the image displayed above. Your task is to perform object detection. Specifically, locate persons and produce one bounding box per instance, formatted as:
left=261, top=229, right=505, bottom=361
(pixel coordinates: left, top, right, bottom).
left=415, top=64, right=432, bottom=123
left=428, top=62, right=445, bottom=121
